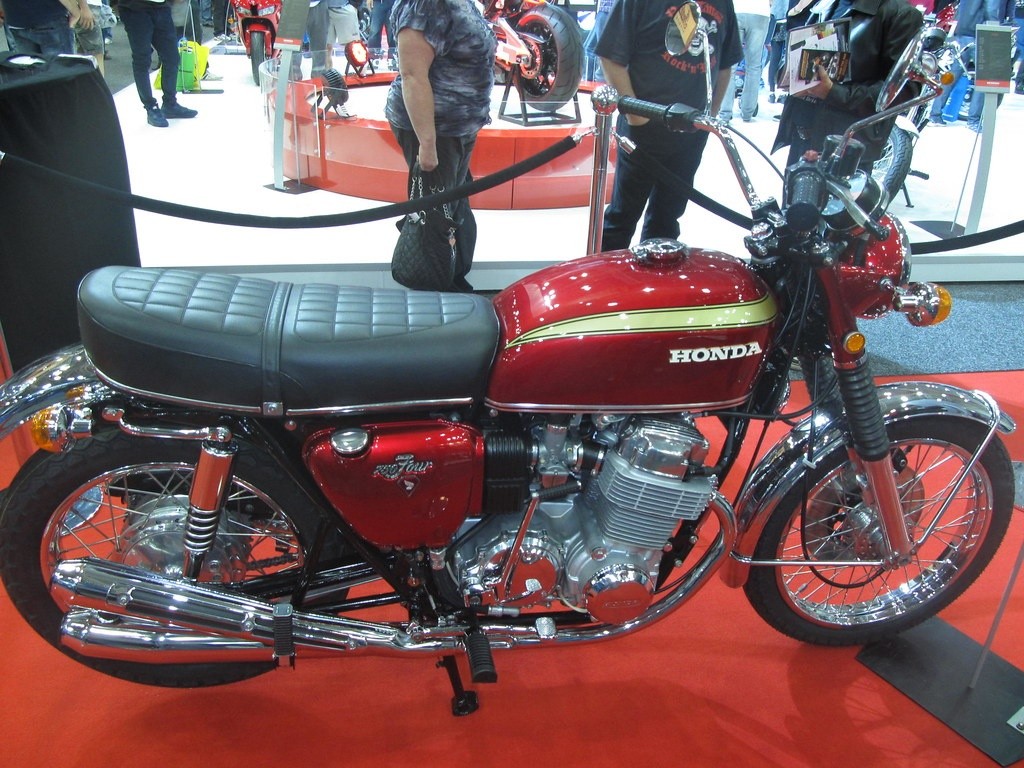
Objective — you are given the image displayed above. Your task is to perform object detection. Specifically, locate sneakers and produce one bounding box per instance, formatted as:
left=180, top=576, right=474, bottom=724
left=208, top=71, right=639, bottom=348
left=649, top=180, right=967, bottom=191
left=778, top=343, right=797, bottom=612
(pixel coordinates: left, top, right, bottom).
left=161, top=103, right=198, bottom=118
left=147, top=109, right=168, bottom=127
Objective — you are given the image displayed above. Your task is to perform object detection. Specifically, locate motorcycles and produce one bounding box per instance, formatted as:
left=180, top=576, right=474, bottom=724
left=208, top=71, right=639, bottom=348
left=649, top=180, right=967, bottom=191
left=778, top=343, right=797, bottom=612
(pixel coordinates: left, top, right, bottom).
left=870, top=18, right=974, bottom=208
left=479, top=0, right=586, bottom=112
left=0, top=66, right=1017, bottom=713
left=956, top=20, right=1020, bottom=121
left=230, top=0, right=282, bottom=84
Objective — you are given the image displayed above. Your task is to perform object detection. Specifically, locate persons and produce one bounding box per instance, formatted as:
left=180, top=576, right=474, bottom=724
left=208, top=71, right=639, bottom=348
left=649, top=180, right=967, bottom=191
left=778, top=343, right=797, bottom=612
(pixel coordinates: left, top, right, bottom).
left=200, top=0, right=232, bottom=35
left=117, top=0, right=198, bottom=127
left=770, top=0, right=925, bottom=211
left=298, top=1, right=395, bottom=74
left=769, top=0, right=790, bottom=103
left=75, top=0, right=109, bottom=79
left=716, top=0, right=770, bottom=124
left=593, top=0, right=746, bottom=252
left=385, top=0, right=499, bottom=292
left=2, top=0, right=82, bottom=55
left=930, top=0, right=1024, bottom=134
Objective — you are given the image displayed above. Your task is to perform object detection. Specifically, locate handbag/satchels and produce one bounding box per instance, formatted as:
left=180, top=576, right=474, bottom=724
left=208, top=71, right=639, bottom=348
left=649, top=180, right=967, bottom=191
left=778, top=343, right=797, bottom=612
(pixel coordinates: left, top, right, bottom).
left=391, top=162, right=459, bottom=290
left=154, top=38, right=209, bottom=93
